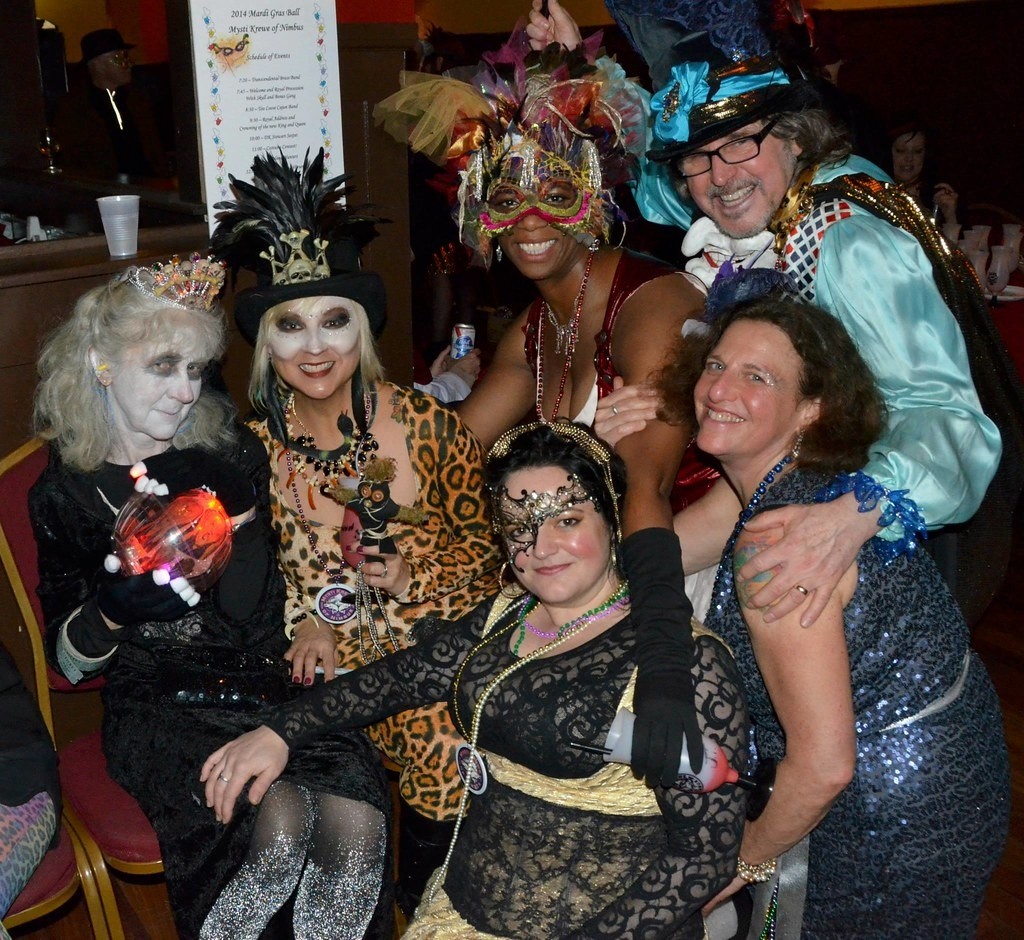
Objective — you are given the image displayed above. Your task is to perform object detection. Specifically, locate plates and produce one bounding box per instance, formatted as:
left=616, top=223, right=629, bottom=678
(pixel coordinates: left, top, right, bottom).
left=983, top=285, right=1024, bottom=301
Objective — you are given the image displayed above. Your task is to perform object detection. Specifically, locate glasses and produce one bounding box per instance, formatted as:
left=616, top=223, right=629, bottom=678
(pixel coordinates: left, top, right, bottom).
left=675, top=113, right=782, bottom=179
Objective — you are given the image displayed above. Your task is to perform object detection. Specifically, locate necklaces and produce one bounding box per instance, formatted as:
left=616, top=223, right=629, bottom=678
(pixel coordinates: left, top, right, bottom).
left=280, top=390, right=400, bottom=665
left=428, top=578, right=631, bottom=899
left=536, top=252, right=594, bottom=423
left=711, top=457, right=791, bottom=607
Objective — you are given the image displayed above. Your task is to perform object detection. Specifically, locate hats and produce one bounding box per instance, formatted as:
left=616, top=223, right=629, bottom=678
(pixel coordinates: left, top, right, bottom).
left=77, top=28, right=134, bottom=65
left=210, top=145, right=387, bottom=349
left=604, top=0, right=817, bottom=164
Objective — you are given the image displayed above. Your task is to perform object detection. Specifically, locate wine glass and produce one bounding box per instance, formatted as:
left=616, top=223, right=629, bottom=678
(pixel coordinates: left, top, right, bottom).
left=940, top=221, right=1022, bottom=307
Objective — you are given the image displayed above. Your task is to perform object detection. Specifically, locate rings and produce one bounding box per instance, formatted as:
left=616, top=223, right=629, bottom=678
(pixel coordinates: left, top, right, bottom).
left=944, top=189, right=952, bottom=194
left=793, top=584, right=810, bottom=596
left=219, top=774, right=230, bottom=783
left=612, top=406, right=619, bottom=416
left=381, top=562, right=387, bottom=578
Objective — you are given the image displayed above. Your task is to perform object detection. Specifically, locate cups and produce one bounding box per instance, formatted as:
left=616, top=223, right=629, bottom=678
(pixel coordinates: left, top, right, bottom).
left=97, top=194, right=142, bottom=257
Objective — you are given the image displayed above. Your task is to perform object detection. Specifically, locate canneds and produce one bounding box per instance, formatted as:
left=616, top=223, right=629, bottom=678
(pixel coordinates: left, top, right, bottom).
left=450, top=322, right=476, bottom=360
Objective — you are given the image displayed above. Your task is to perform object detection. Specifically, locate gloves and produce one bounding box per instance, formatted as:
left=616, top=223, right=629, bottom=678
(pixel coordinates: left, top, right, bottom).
left=95, top=554, right=200, bottom=627
left=617, top=527, right=704, bottom=790
left=129, top=447, right=256, bottom=517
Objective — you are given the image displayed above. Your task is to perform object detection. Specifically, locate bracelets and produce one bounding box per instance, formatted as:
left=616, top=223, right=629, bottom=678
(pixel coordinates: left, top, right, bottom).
left=736, top=857, right=777, bottom=883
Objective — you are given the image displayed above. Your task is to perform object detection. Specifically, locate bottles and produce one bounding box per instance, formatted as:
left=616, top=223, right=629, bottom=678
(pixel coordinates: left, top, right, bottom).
left=33, top=123, right=65, bottom=174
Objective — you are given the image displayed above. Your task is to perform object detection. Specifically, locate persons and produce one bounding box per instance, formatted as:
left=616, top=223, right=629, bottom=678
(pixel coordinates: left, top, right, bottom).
left=26, top=253, right=393, bottom=940
left=62, top=29, right=151, bottom=188
left=200, top=424, right=751, bottom=940
left=0, top=645, right=60, bottom=940
left=205, top=149, right=509, bottom=916
left=594, top=293, right=1012, bottom=940
left=370, top=0, right=1012, bottom=791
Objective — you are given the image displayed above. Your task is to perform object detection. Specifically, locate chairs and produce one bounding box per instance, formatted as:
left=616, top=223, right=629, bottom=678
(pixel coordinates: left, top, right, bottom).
left=0, top=428, right=164, bottom=940
left=3, top=813, right=111, bottom=940
